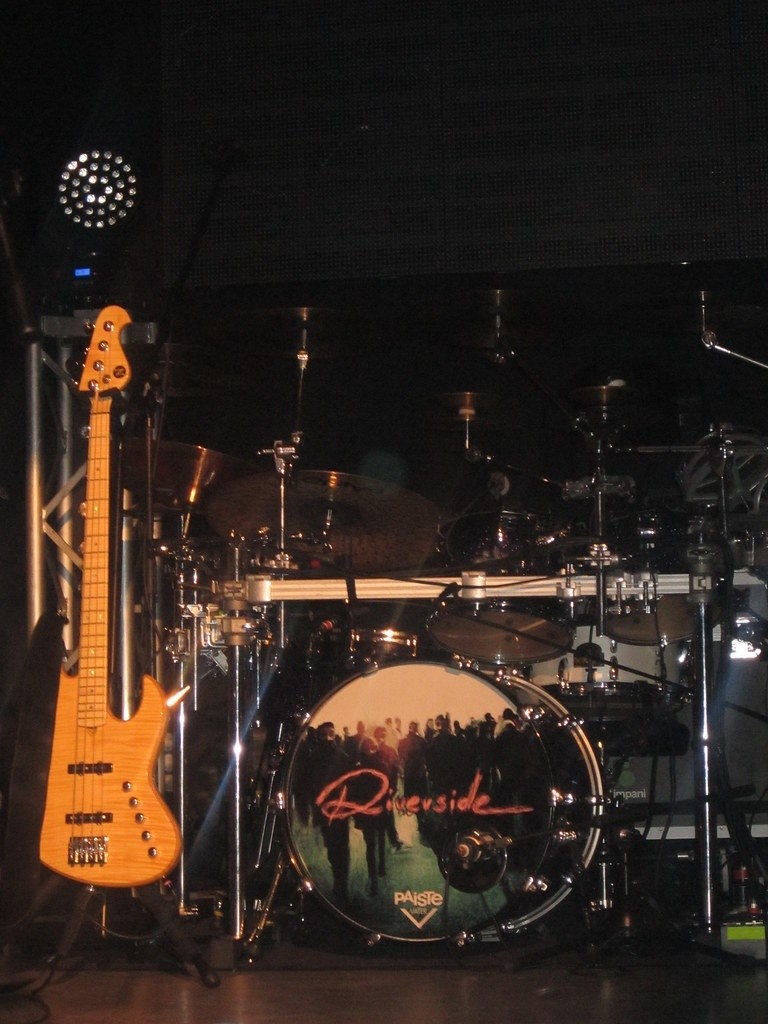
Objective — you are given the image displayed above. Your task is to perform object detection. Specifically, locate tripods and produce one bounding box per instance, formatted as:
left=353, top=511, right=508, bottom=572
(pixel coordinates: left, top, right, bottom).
left=6, top=381, right=768, bottom=993
